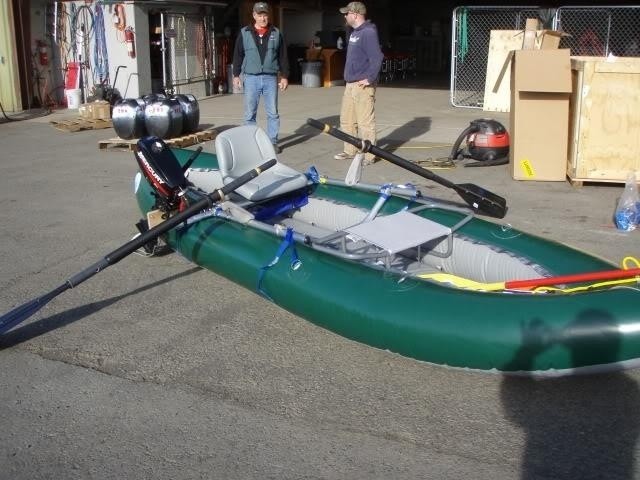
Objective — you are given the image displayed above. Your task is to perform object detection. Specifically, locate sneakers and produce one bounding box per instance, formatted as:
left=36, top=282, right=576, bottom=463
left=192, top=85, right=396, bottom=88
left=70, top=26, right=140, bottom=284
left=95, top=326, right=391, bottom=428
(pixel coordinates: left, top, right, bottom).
left=334, top=152, right=355, bottom=160
left=273, top=143, right=280, bottom=154
left=362, top=159, right=375, bottom=166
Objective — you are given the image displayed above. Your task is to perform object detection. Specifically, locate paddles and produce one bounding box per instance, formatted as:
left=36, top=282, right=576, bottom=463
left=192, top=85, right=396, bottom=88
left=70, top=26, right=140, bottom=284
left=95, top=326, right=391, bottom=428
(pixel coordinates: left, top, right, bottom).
left=0, top=159, right=276, bottom=333
left=306, top=118, right=508, bottom=218
left=420, top=268, right=639, bottom=292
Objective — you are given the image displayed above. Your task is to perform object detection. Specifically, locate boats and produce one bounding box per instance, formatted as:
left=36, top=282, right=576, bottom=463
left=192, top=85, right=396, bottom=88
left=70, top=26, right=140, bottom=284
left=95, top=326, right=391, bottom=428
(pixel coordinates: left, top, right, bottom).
left=133, top=135, right=640, bottom=377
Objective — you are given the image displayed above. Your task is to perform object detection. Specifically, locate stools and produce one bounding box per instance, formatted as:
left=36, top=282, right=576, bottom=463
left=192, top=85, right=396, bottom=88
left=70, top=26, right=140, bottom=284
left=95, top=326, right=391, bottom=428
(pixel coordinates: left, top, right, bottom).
left=339, top=210, right=453, bottom=269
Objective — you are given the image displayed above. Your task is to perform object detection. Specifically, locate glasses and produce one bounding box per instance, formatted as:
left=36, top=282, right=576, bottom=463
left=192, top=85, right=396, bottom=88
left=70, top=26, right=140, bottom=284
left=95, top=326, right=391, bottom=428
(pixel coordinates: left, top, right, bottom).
left=345, top=12, right=353, bottom=15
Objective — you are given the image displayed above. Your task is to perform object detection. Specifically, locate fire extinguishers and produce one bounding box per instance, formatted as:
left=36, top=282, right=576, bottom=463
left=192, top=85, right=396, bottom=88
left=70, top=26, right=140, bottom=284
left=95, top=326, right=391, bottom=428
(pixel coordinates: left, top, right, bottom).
left=125, top=26, right=136, bottom=57
left=39, top=39, right=48, bottom=65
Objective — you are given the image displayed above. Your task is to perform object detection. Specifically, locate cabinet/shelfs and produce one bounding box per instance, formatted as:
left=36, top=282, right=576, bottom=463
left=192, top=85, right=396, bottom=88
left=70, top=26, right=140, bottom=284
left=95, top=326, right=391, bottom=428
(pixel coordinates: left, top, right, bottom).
left=567, top=56, right=640, bottom=186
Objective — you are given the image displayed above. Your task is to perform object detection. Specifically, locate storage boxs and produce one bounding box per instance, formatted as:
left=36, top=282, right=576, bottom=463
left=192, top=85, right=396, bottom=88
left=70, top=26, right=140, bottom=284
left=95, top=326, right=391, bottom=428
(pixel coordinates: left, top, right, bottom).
left=492, top=17, right=574, bottom=182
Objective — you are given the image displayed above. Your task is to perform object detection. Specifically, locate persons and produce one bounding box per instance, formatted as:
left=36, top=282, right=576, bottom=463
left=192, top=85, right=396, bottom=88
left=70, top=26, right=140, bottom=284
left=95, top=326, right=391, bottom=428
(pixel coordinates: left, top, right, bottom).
left=232, top=2, right=290, bottom=154
left=333, top=1, right=384, bottom=166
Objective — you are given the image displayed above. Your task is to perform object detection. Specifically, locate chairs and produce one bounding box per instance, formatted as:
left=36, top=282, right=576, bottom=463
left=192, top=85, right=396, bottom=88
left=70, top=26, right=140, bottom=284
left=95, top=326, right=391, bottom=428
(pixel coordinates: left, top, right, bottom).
left=214, top=124, right=308, bottom=201
left=381, top=55, right=417, bottom=81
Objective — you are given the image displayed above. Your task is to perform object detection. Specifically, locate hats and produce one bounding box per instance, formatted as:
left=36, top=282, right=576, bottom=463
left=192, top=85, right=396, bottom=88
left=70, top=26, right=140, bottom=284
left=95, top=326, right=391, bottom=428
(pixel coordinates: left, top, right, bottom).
left=253, top=3, right=270, bottom=13
left=339, top=2, right=367, bottom=14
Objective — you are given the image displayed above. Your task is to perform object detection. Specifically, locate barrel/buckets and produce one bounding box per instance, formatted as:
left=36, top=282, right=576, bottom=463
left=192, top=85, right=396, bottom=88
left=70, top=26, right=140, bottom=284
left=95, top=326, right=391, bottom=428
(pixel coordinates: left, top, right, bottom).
left=64, top=88, right=82, bottom=109
left=306, top=39, right=321, bottom=61
